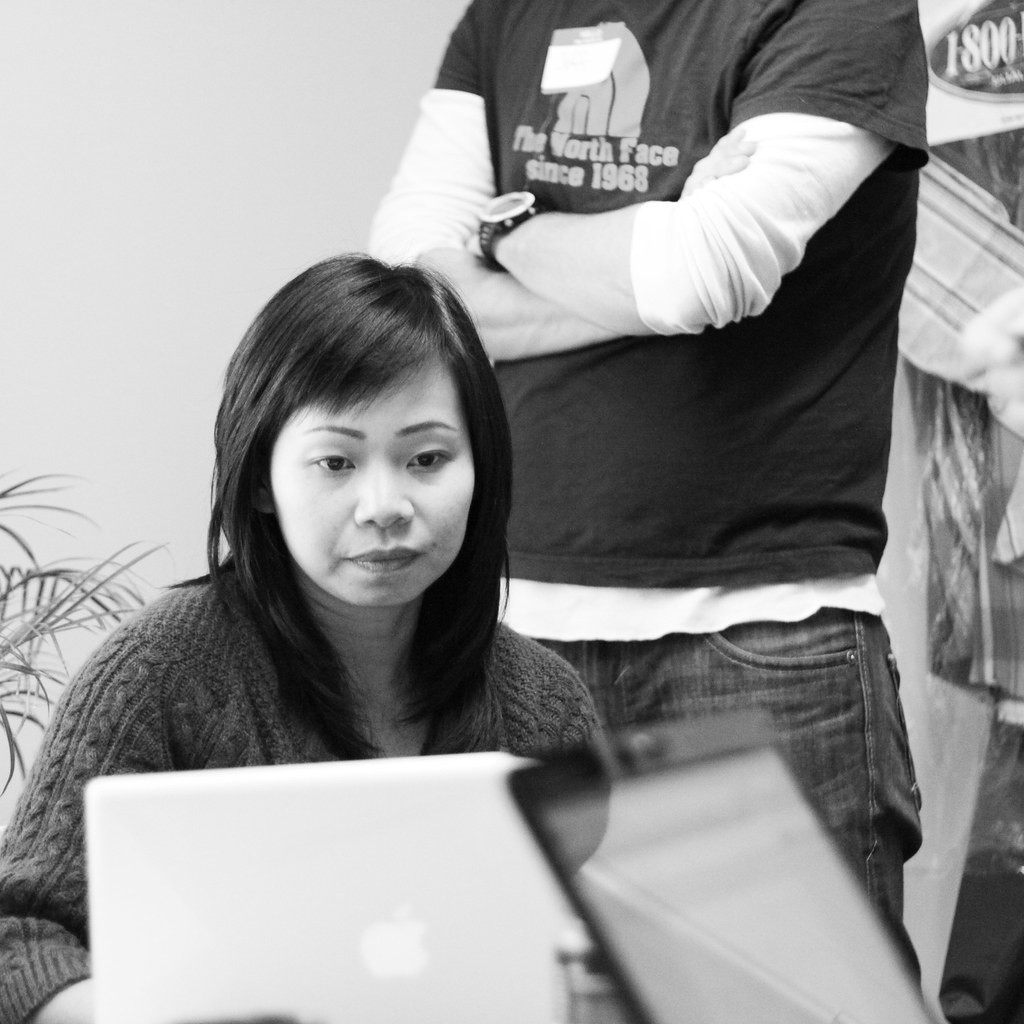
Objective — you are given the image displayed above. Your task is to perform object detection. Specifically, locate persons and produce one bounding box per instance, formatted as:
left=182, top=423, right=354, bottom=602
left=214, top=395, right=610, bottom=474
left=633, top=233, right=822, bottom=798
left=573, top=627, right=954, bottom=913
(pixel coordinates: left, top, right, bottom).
left=897, top=0, right=1024, bottom=1024
left=366, top=0, right=935, bottom=1024
left=0, top=251, right=607, bottom=1024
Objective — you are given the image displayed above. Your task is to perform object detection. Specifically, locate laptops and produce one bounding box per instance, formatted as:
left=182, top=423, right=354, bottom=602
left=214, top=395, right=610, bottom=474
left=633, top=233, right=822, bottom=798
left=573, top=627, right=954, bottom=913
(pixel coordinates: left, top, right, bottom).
left=86, top=748, right=570, bottom=1024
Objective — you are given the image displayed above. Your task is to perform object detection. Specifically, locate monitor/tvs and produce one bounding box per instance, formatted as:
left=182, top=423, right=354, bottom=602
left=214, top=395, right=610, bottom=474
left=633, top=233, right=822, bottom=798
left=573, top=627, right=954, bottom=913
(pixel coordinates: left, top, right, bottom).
left=505, top=708, right=934, bottom=1024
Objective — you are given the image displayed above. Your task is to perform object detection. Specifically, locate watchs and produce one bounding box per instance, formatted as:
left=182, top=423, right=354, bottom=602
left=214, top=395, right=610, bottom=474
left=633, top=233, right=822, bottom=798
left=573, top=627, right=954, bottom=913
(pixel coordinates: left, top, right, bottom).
left=475, top=190, right=558, bottom=273
